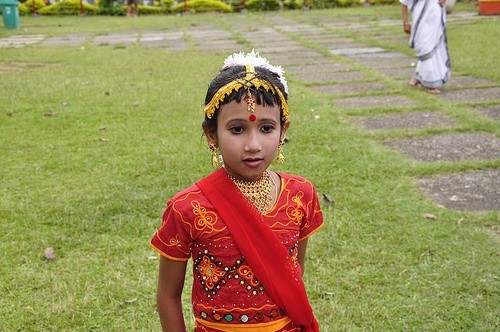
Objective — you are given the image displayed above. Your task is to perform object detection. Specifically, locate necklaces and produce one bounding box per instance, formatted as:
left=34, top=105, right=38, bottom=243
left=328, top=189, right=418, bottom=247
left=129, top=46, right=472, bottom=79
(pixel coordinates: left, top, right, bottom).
left=227, top=172, right=272, bottom=214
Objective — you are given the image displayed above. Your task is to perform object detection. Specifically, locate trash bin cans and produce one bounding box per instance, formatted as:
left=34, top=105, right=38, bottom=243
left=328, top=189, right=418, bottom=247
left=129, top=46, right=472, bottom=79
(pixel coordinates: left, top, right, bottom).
left=0, top=0, right=20, bottom=29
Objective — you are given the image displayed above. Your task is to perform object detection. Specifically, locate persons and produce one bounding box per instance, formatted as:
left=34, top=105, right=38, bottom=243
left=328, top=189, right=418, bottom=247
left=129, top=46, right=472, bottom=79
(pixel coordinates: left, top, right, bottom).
left=151, top=53, right=323, bottom=332
left=401, top=0, right=450, bottom=93
